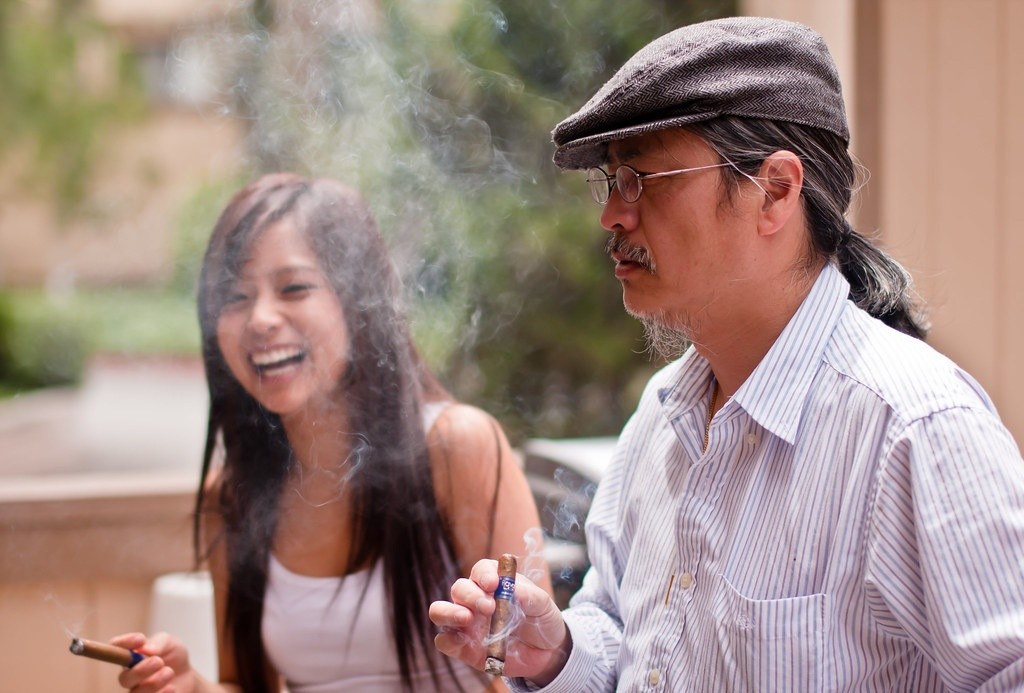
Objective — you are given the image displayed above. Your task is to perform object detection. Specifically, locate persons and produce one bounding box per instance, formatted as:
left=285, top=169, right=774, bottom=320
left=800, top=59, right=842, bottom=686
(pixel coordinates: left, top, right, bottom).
left=427, top=14, right=1024, bottom=692
left=109, top=166, right=556, bottom=693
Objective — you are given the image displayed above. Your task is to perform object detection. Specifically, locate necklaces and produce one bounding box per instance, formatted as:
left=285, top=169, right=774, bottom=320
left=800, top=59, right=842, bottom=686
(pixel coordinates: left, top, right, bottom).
left=291, top=477, right=349, bottom=511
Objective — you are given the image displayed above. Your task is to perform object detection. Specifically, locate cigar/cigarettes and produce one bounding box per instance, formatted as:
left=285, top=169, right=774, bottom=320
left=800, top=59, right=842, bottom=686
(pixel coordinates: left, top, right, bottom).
left=68, top=638, right=150, bottom=670
left=484, top=553, right=518, bottom=677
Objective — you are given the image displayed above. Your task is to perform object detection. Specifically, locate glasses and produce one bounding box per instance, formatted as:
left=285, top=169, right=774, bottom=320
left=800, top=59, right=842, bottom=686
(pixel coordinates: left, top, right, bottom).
left=586, top=161, right=739, bottom=205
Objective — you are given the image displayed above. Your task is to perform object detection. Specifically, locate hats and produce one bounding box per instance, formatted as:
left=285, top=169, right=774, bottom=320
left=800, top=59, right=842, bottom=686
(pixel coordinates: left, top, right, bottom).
left=552, top=16, right=850, bottom=172
